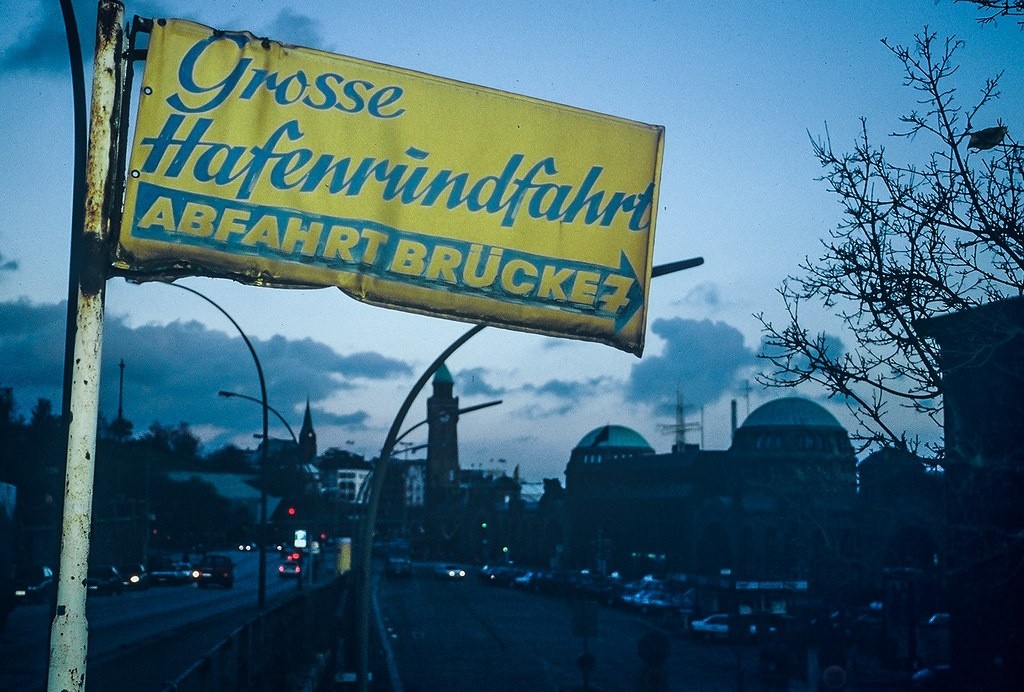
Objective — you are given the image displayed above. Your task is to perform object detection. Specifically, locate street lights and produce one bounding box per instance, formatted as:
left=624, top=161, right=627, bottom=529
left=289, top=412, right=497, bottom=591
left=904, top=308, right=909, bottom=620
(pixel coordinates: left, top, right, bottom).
left=357, top=256, right=708, bottom=692
left=217, top=389, right=305, bottom=587
left=126, top=276, right=271, bottom=611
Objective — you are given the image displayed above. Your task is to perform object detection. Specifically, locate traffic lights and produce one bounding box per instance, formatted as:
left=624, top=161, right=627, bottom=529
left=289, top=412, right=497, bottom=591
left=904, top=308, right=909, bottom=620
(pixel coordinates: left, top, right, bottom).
left=481, top=520, right=489, bottom=530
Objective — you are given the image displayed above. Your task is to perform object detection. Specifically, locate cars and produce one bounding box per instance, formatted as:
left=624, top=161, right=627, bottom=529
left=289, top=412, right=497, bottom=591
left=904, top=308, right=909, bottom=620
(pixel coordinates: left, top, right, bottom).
left=8, top=565, right=55, bottom=602
left=85, top=558, right=195, bottom=593
left=278, top=544, right=305, bottom=578
left=380, top=548, right=682, bottom=615
left=690, top=613, right=760, bottom=639
left=193, top=555, right=237, bottom=589
